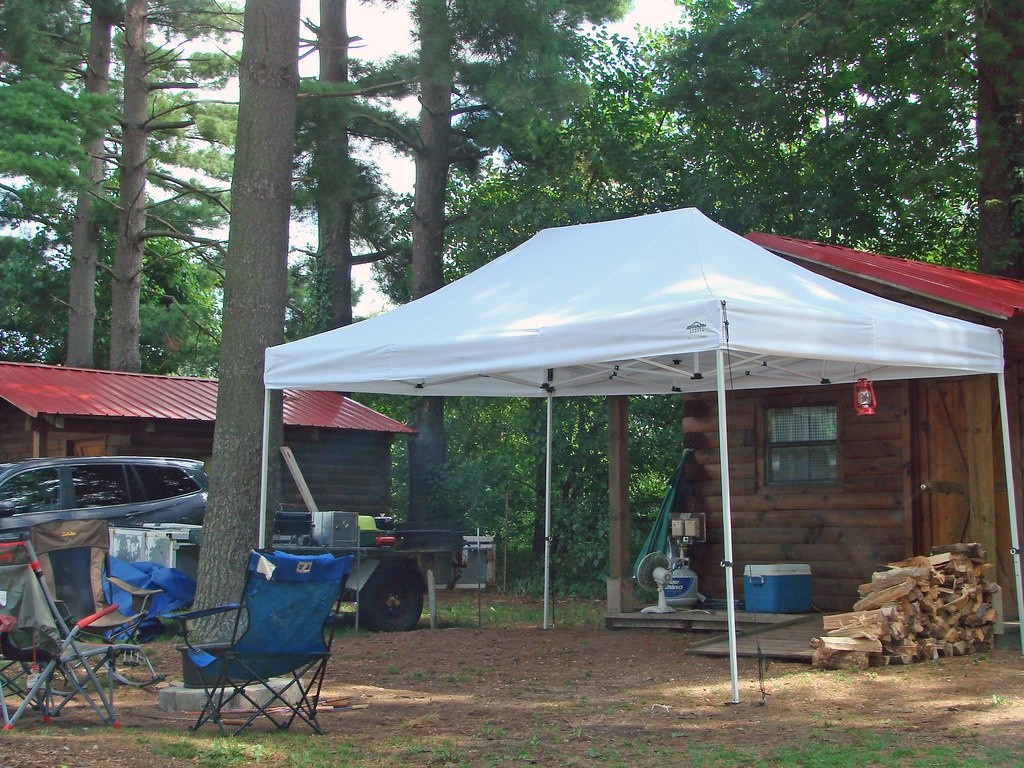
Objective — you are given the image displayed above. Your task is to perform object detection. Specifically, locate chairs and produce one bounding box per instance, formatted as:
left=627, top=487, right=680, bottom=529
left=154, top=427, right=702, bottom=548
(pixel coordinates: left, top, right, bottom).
left=161, top=550, right=355, bottom=737
left=0, top=532, right=122, bottom=730
left=31, top=519, right=166, bottom=696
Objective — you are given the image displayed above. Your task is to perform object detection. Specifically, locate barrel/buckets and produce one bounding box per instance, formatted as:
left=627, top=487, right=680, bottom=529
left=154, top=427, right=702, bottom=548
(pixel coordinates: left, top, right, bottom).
left=743, top=564, right=813, bottom=612
left=272, top=511, right=315, bottom=545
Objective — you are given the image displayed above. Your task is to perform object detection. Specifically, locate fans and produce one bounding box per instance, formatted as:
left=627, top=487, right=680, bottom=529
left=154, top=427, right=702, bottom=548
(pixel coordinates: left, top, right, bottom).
left=636, top=551, right=676, bottom=613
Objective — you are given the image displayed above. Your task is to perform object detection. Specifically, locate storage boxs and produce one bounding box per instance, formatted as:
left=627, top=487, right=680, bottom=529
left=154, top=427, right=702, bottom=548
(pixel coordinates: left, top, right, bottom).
left=743, top=564, right=812, bottom=614
left=313, top=512, right=358, bottom=546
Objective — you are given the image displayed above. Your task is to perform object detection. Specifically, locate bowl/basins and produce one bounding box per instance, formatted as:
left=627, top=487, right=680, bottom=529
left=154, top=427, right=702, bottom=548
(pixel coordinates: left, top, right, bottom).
left=358, top=516, right=379, bottom=530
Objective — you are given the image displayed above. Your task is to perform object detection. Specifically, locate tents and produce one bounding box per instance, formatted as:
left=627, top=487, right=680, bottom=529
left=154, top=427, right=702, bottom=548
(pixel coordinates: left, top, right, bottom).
left=256, top=206, right=1022, bottom=707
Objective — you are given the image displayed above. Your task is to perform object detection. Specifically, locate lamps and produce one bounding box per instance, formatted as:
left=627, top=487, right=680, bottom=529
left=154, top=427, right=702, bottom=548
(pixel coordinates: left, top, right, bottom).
left=853, top=364, right=876, bottom=416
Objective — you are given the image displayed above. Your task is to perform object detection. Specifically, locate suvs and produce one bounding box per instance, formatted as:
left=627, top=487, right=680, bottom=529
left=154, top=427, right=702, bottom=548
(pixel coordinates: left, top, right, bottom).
left=0, top=456, right=210, bottom=559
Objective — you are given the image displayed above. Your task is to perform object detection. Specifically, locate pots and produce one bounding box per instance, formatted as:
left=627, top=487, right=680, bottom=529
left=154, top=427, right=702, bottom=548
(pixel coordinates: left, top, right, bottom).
left=374, top=513, right=394, bottom=530
